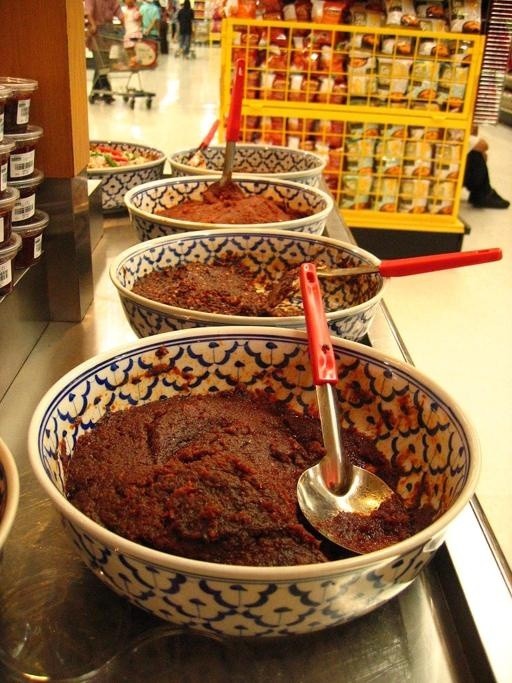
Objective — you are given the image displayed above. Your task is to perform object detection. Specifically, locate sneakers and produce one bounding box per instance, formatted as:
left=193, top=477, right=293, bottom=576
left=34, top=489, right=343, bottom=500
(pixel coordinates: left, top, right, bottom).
left=474, top=190, right=510, bottom=207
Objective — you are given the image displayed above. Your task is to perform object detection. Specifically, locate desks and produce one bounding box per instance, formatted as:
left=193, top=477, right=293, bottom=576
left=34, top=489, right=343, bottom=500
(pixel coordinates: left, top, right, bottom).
left=2, top=173, right=511, bottom=683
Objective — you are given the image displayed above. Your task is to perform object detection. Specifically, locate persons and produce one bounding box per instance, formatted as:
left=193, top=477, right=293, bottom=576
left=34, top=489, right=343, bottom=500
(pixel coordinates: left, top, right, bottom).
left=461, top=134, right=511, bottom=208
left=117, top=0, right=176, bottom=67
left=176, top=0, right=194, bottom=58
left=85, top=0, right=126, bottom=104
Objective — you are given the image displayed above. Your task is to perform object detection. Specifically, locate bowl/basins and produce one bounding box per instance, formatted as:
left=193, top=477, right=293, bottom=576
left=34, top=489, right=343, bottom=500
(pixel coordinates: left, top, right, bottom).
left=106, top=228, right=393, bottom=342
left=86, top=138, right=337, bottom=237
left=21, top=324, right=485, bottom=650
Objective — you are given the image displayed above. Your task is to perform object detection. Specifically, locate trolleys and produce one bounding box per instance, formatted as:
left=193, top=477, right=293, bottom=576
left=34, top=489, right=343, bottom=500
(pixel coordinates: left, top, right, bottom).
left=85, top=28, right=160, bottom=109
